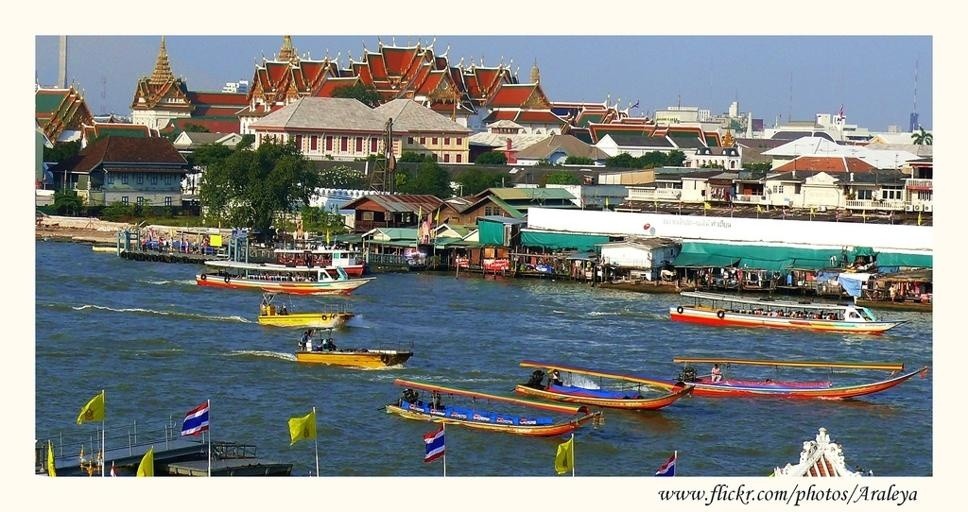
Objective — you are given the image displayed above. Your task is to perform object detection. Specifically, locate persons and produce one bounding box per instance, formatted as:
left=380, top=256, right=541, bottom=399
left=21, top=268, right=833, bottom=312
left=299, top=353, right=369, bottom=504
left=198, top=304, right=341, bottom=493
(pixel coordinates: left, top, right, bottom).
left=138, top=216, right=931, bottom=387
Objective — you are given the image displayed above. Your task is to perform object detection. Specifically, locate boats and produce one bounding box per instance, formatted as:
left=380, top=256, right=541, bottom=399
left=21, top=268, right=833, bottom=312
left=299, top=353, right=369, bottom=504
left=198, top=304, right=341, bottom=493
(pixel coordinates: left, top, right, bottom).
left=383, top=377, right=607, bottom=439
left=666, top=286, right=910, bottom=337
left=274, top=242, right=369, bottom=278
left=190, top=256, right=368, bottom=302
left=671, top=347, right=928, bottom=404
left=290, top=344, right=410, bottom=370
left=255, top=287, right=356, bottom=330
left=511, top=355, right=699, bottom=412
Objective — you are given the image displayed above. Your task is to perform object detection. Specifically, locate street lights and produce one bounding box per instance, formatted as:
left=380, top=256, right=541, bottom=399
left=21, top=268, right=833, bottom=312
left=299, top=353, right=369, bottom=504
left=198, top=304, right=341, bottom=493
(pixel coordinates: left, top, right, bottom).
left=447, top=180, right=464, bottom=196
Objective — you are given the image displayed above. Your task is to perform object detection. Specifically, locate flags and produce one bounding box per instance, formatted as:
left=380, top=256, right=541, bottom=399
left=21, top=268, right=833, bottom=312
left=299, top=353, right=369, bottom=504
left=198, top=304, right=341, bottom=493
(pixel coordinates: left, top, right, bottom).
left=77, top=393, right=104, bottom=424
left=288, top=412, right=317, bottom=447
left=655, top=455, right=676, bottom=476
left=47, top=444, right=57, bottom=477
left=555, top=437, right=573, bottom=475
left=423, top=426, right=445, bottom=462
left=180, top=401, right=209, bottom=436
left=137, top=450, right=155, bottom=477
